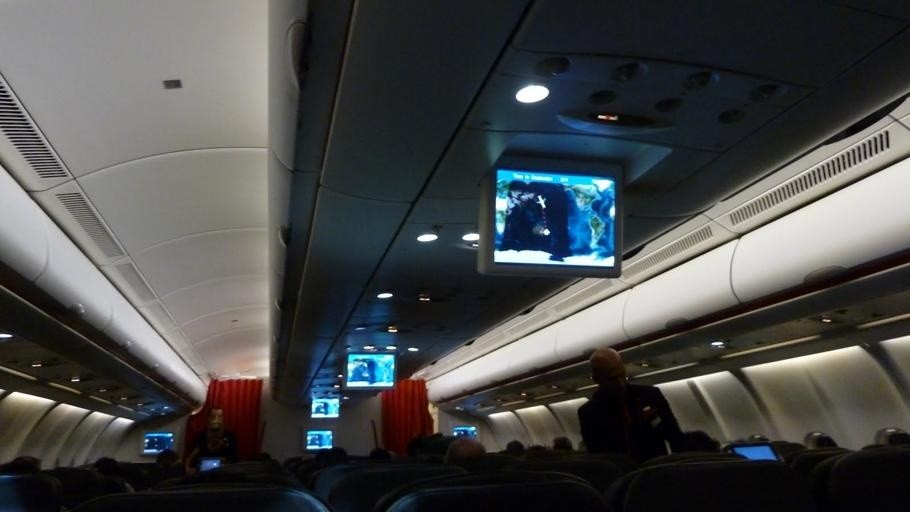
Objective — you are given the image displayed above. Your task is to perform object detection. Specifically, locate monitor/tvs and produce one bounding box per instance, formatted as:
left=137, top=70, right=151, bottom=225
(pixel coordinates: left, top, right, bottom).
left=448, top=424, right=481, bottom=440
left=477, top=152, right=625, bottom=276
left=304, top=428, right=333, bottom=450
left=310, top=393, right=341, bottom=419
left=140, top=429, right=179, bottom=456
left=343, top=351, right=397, bottom=389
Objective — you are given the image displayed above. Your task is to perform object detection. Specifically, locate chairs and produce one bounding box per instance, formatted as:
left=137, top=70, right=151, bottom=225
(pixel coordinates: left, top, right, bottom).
left=788, top=444, right=906, bottom=512
left=297, top=450, right=617, bottom=512
left=0, top=463, right=328, bottom=512
left=613, top=461, right=814, bottom=512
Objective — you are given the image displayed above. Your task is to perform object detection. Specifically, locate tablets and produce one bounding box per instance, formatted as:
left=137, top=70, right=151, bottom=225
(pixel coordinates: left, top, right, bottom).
left=196, top=458, right=222, bottom=471
left=729, top=443, right=780, bottom=462
